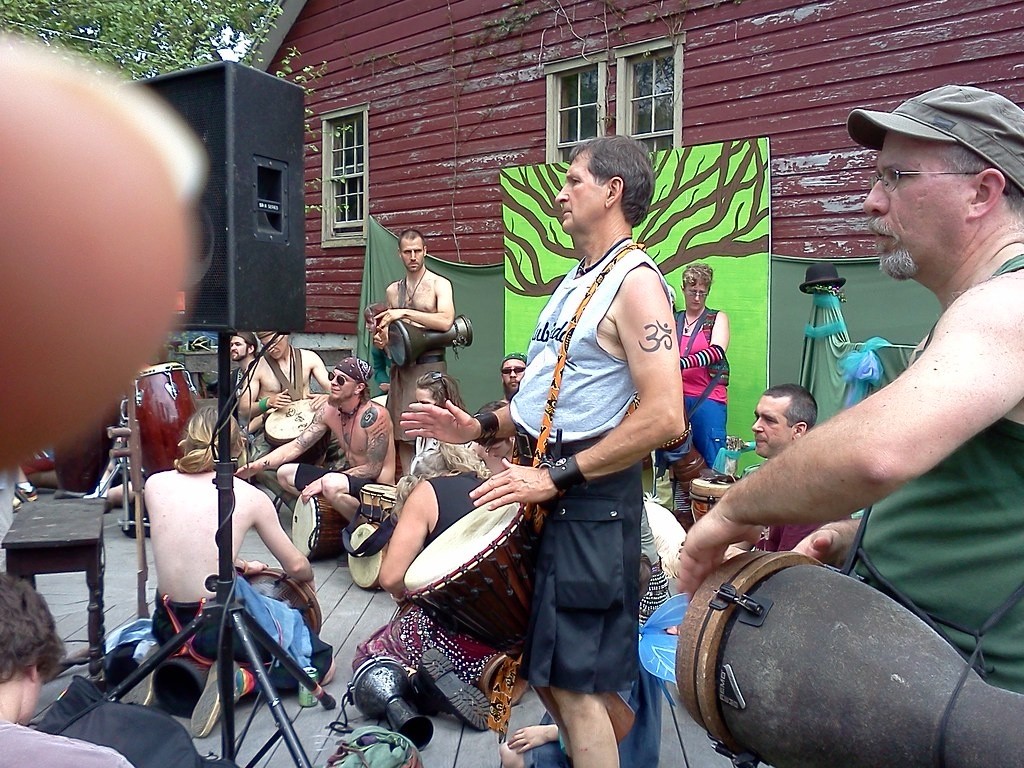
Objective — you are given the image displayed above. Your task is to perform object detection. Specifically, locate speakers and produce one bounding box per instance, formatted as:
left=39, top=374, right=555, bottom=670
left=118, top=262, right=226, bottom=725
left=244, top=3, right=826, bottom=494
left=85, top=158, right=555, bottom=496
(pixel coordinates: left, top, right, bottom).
left=122, top=61, right=308, bottom=334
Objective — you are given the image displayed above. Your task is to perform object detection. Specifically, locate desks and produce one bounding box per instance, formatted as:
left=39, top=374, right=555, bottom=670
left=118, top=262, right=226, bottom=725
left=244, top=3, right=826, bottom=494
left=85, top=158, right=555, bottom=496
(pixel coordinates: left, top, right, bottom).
left=1, top=498, right=108, bottom=694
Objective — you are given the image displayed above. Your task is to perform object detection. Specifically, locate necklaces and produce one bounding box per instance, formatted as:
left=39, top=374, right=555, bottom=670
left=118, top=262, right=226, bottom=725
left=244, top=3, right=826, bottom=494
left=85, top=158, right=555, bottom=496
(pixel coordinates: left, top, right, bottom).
left=577, top=236, right=632, bottom=274
left=285, top=346, right=292, bottom=384
left=405, top=269, right=427, bottom=300
left=338, top=401, right=361, bottom=465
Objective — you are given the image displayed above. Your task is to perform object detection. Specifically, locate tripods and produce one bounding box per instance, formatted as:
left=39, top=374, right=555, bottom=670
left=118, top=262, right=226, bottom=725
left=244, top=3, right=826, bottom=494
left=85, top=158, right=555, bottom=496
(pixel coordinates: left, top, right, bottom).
left=102, top=330, right=336, bottom=768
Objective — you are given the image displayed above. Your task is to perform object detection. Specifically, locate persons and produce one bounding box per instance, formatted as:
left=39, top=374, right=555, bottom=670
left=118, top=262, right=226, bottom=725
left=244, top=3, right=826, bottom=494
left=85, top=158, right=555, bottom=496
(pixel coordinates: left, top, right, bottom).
left=499, top=352, right=527, bottom=404
left=227, top=326, right=341, bottom=509
left=0, top=393, right=136, bottom=551
left=415, top=371, right=470, bottom=455
left=678, top=84, right=1024, bottom=693
left=374, top=227, right=455, bottom=473
left=468, top=400, right=515, bottom=476
left=500, top=551, right=661, bottom=768
left=729, top=383, right=852, bottom=552
left=353, top=441, right=504, bottom=731
left=234, top=356, right=396, bottom=568
left=639, top=504, right=673, bottom=629
left=0, top=571, right=135, bottom=768
left=653, top=262, right=730, bottom=468
left=400, top=133, right=686, bottom=768
left=144, top=405, right=335, bottom=736
left=364, top=303, right=395, bottom=393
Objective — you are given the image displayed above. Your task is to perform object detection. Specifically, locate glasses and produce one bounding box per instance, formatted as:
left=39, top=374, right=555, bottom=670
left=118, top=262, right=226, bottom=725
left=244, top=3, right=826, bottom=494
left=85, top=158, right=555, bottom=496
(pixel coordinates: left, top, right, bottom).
left=423, top=371, right=448, bottom=394
left=501, top=367, right=526, bottom=374
left=869, top=168, right=1010, bottom=197
left=682, top=288, right=708, bottom=298
left=327, top=371, right=354, bottom=386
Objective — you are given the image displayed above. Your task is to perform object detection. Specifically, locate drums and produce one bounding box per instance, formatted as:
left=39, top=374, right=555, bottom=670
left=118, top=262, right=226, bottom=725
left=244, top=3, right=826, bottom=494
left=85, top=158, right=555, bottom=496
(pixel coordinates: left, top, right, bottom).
left=290, top=492, right=350, bottom=563
left=658, top=406, right=711, bottom=498
left=360, top=481, right=398, bottom=520
left=133, top=362, right=197, bottom=477
left=347, top=522, right=391, bottom=590
left=690, top=477, right=734, bottom=524
left=674, top=549, right=1024, bottom=768
left=242, top=567, right=324, bottom=637
left=264, top=397, right=330, bottom=468
left=401, top=496, right=637, bottom=761
left=349, top=657, right=435, bottom=752
left=381, top=491, right=404, bottom=522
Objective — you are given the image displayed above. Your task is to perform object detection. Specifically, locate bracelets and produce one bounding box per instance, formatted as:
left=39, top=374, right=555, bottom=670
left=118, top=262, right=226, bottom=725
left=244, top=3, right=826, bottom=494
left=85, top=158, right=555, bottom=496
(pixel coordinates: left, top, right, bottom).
left=259, top=396, right=269, bottom=412
left=474, top=411, right=500, bottom=440
left=548, top=455, right=588, bottom=493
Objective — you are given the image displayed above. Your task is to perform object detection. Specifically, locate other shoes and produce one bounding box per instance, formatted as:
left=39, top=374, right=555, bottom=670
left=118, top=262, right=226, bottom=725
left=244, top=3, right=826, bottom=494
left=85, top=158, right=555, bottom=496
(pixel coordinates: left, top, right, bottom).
left=190, top=659, right=244, bottom=739
left=413, top=648, right=496, bottom=732
left=121, top=658, right=160, bottom=711
left=336, top=551, right=349, bottom=567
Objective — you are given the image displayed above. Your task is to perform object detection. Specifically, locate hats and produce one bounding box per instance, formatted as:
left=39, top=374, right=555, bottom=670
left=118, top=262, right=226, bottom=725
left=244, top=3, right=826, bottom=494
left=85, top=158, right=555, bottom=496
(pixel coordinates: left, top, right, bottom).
left=235, top=331, right=259, bottom=351
left=847, top=84, right=1024, bottom=187
left=799, top=263, right=846, bottom=294
